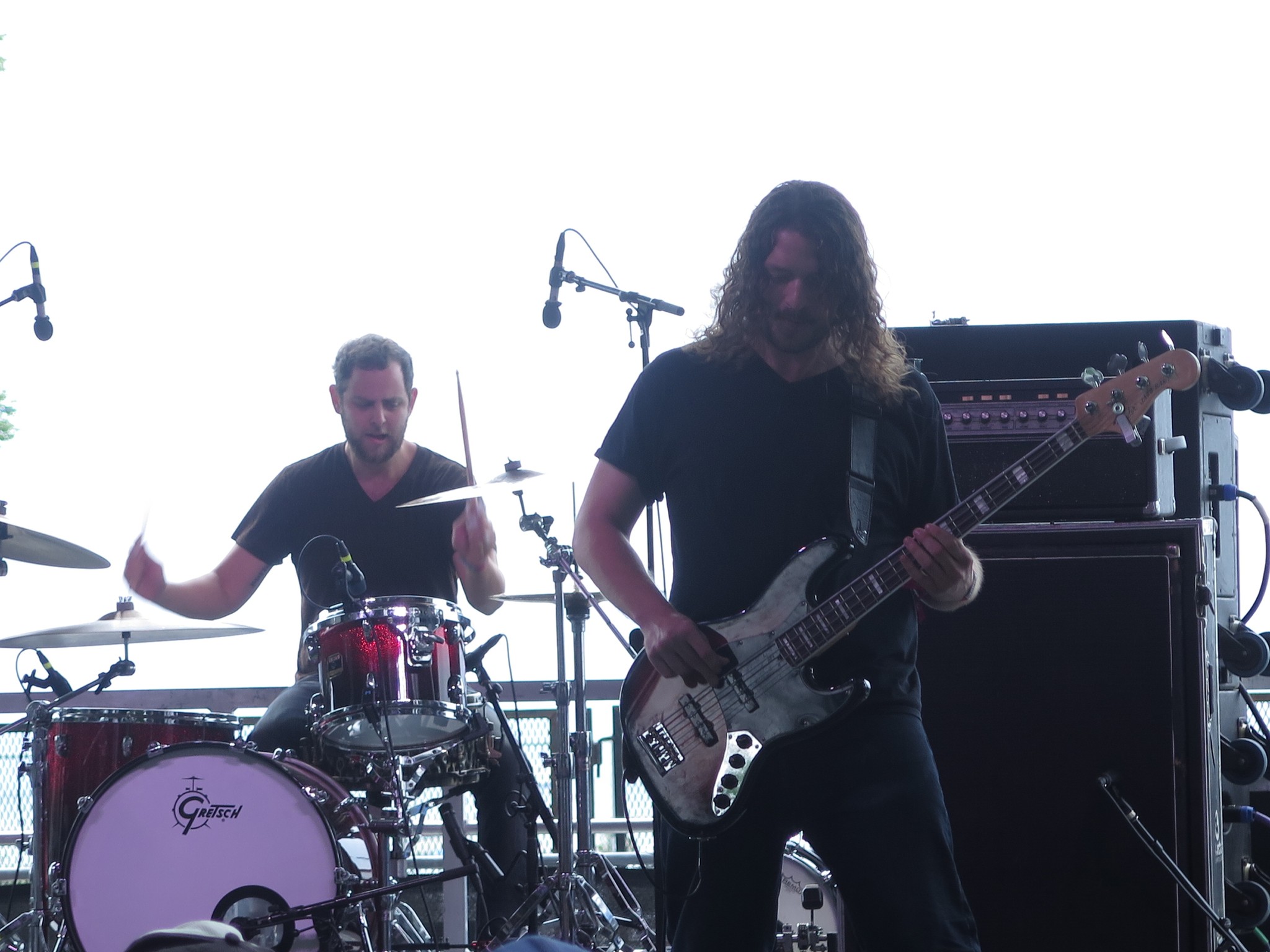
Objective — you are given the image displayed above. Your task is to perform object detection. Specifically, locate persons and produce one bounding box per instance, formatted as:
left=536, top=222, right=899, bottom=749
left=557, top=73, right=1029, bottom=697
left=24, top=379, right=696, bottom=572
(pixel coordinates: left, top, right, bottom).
left=123, top=333, right=539, bottom=952
left=572, top=180, right=984, bottom=952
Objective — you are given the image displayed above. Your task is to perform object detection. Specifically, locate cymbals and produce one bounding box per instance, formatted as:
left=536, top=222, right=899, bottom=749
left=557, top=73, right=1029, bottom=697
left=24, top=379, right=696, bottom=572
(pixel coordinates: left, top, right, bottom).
left=395, top=467, right=562, bottom=508
left=0, top=514, right=111, bottom=570
left=487, top=591, right=608, bottom=607
left=0, top=612, right=266, bottom=649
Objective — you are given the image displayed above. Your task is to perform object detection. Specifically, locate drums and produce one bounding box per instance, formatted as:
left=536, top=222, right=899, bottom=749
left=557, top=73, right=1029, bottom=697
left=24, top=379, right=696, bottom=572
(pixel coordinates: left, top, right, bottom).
left=777, top=839, right=849, bottom=952
left=329, top=691, right=496, bottom=792
left=29, top=699, right=245, bottom=922
left=59, top=739, right=386, bottom=952
left=306, top=595, right=476, bottom=752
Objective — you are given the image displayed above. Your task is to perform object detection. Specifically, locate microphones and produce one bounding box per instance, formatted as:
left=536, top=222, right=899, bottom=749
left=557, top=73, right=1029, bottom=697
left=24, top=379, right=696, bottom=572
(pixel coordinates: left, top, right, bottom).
left=1092, top=771, right=1112, bottom=789
left=30, top=245, right=53, bottom=340
left=336, top=540, right=367, bottom=597
left=439, top=802, right=483, bottom=895
left=542, top=232, right=565, bottom=328
left=37, top=650, right=72, bottom=697
left=464, top=634, right=501, bottom=671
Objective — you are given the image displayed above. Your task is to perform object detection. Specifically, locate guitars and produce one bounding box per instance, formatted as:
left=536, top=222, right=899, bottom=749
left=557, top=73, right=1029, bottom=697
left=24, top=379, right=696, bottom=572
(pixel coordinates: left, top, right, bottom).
left=618, top=327, right=1205, bottom=838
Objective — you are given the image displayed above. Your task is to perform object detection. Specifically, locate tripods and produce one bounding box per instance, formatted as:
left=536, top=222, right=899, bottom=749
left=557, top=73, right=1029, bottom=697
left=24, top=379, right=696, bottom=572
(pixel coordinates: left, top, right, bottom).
left=476, top=514, right=655, bottom=952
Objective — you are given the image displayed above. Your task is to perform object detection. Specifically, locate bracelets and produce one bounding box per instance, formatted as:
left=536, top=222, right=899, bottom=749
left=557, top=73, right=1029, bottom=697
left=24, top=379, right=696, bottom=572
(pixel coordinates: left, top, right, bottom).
left=959, top=570, right=977, bottom=602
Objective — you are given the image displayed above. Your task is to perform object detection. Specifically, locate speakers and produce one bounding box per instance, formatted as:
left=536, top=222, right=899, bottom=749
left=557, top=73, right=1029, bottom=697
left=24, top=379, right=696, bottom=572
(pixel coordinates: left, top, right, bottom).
left=882, top=320, right=1260, bottom=952
